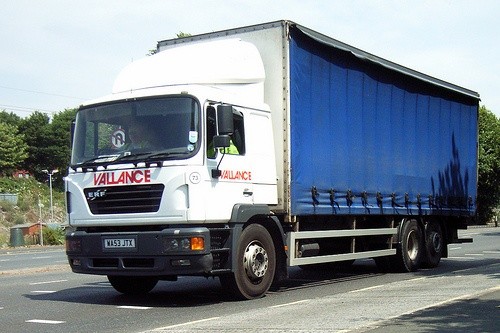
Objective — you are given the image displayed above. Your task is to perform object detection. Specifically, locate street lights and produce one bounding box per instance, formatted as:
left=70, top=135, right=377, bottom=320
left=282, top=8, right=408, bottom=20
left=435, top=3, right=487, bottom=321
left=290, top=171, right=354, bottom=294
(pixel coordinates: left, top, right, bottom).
left=41, top=170, right=59, bottom=225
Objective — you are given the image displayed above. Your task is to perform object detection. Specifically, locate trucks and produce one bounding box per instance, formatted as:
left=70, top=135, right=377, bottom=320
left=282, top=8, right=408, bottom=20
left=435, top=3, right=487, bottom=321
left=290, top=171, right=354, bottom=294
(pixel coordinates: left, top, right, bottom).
left=65, top=19, right=481, bottom=301
